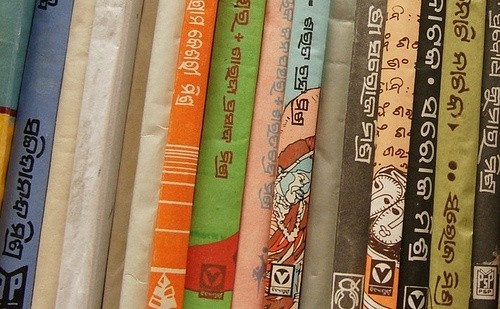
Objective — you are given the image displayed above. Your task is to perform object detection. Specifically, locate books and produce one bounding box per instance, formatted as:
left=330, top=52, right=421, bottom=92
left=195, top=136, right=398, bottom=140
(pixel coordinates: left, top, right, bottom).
left=0, top=0, right=500, bottom=309
